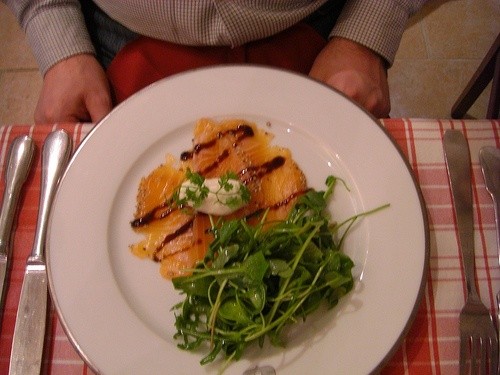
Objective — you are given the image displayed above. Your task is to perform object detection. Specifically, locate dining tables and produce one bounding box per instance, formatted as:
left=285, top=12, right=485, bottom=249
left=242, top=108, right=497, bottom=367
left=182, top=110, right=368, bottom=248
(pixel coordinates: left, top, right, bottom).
left=0, top=117, right=500, bottom=375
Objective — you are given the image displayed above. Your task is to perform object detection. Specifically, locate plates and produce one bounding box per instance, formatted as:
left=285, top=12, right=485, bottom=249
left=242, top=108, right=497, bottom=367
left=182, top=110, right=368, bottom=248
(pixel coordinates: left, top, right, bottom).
left=46, top=66, right=429, bottom=375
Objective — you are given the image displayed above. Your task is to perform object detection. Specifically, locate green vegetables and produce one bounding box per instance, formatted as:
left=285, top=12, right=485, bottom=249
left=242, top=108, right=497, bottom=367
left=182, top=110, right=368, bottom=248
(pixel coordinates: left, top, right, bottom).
left=166, top=160, right=392, bottom=375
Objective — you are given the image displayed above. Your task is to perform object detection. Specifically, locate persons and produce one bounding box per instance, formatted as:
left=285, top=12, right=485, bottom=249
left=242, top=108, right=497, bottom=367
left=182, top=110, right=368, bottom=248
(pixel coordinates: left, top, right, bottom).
left=0, top=0, right=431, bottom=125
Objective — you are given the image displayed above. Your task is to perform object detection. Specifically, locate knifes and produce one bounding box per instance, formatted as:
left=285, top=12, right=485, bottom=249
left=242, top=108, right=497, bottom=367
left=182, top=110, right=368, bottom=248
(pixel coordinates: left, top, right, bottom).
left=8, top=129, right=71, bottom=375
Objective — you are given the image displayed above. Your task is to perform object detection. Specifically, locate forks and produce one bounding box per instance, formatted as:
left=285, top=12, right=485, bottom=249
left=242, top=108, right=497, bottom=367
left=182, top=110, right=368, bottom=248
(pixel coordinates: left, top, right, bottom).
left=442, top=130, right=498, bottom=375
